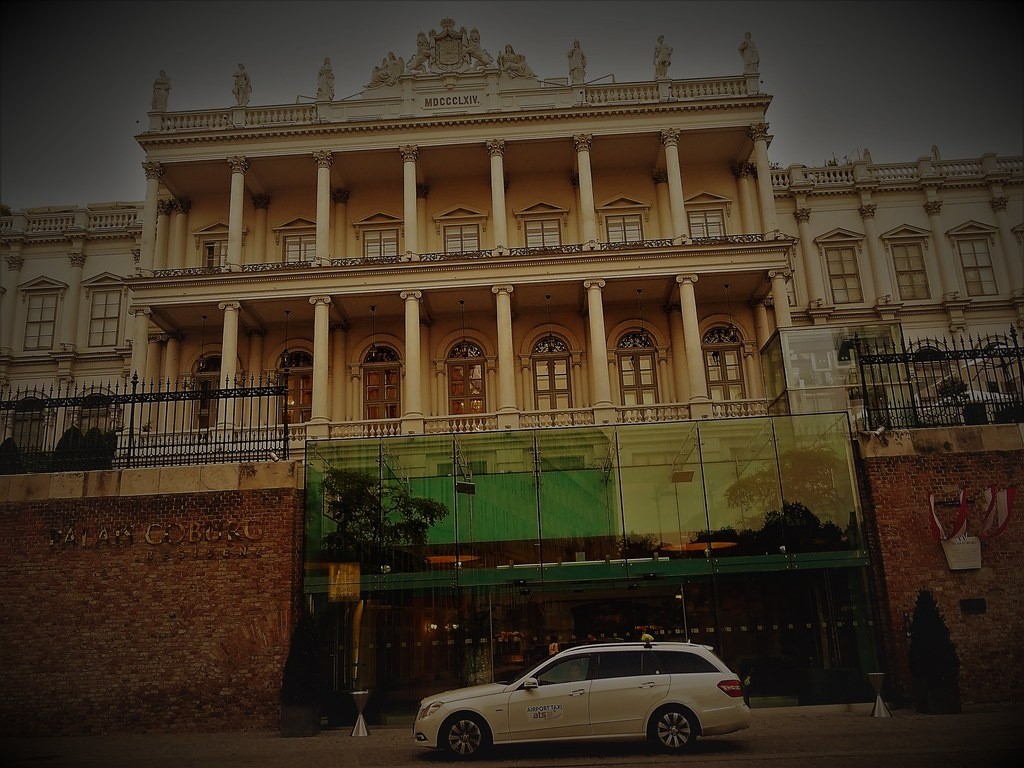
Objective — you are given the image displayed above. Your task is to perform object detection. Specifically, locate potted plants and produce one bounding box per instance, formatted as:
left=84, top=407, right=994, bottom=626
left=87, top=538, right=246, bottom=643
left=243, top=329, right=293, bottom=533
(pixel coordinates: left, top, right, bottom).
left=281, top=602, right=325, bottom=738
left=907, top=585, right=963, bottom=715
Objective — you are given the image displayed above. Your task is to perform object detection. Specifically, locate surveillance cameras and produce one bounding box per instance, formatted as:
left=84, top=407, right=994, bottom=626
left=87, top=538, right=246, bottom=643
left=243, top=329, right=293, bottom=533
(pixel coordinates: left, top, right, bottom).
left=874, top=427, right=885, bottom=437
left=270, top=452, right=279, bottom=461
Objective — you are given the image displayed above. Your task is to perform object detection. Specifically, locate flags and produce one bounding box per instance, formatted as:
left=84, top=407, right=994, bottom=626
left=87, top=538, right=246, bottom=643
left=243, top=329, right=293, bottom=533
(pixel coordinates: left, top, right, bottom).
left=927, top=485, right=1016, bottom=541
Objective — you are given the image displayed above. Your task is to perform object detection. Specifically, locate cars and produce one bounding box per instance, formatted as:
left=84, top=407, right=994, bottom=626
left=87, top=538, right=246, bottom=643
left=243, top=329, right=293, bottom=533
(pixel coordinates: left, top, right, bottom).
left=412, top=635, right=751, bottom=761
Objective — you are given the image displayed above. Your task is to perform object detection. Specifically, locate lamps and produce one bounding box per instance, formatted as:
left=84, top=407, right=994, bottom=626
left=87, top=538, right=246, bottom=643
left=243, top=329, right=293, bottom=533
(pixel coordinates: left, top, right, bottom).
left=545, top=295, right=555, bottom=349
left=281, top=311, right=290, bottom=362
left=197, top=315, right=207, bottom=370
left=369, top=306, right=376, bottom=358
left=724, top=282, right=736, bottom=339
left=636, top=289, right=648, bottom=343
left=459, top=301, right=467, bottom=356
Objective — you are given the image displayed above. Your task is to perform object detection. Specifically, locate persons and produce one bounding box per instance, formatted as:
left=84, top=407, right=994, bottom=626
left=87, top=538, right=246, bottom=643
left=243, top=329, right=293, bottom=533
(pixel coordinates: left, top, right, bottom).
left=737, top=32, right=760, bottom=73
left=652, top=34, right=674, bottom=80
left=566, top=38, right=587, bottom=84
left=368, top=16, right=531, bottom=84
left=316, top=57, right=336, bottom=101
left=151, top=70, right=171, bottom=111
left=232, top=64, right=252, bottom=105
